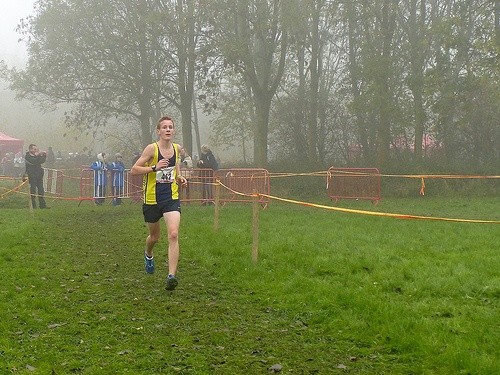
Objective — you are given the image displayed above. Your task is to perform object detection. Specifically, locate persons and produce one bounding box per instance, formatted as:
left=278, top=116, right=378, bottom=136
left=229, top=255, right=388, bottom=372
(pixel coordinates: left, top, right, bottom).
left=130, top=116, right=188, bottom=291
left=111, top=153, right=125, bottom=205
left=92, top=152, right=110, bottom=205
left=179, top=147, right=194, bottom=205
left=197, top=144, right=218, bottom=206
left=25, top=144, right=51, bottom=209
left=2, top=152, right=25, bottom=175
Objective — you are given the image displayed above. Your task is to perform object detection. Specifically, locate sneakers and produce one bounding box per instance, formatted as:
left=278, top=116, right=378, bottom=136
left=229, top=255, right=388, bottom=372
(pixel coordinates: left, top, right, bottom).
left=144, top=250, right=154, bottom=274
left=166, top=275, right=178, bottom=290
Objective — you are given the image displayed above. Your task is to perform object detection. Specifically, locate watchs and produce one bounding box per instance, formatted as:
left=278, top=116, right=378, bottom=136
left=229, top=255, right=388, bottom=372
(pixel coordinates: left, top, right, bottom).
left=151, top=165, right=157, bottom=172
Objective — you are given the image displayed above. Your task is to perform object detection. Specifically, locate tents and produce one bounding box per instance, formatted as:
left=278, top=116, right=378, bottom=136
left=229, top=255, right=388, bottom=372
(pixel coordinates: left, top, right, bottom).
left=0, top=131, right=24, bottom=161
